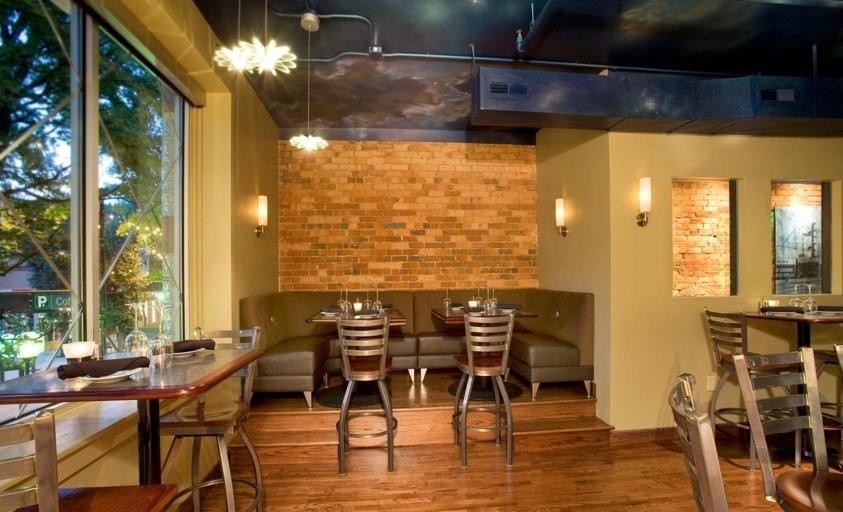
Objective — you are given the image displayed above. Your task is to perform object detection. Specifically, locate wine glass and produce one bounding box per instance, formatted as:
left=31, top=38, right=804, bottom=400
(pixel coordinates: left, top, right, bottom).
left=475, top=285, right=497, bottom=313
left=124, top=301, right=147, bottom=356
left=788, top=282, right=819, bottom=316
left=441, top=284, right=452, bottom=310
left=363, top=285, right=382, bottom=311
left=337, top=285, right=351, bottom=313
left=147, top=301, right=175, bottom=370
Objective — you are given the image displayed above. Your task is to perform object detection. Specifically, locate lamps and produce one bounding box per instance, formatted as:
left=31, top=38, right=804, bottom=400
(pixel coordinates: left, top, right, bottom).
left=213, top=0, right=297, bottom=77
left=287, top=13, right=329, bottom=152
left=254, top=192, right=269, bottom=238
left=554, top=197, right=568, bottom=237
left=636, top=176, right=652, bottom=227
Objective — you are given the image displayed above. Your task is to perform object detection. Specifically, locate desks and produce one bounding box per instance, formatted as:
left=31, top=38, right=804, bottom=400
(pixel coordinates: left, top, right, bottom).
left=0, top=345, right=267, bottom=485
left=741, top=310, right=842, bottom=460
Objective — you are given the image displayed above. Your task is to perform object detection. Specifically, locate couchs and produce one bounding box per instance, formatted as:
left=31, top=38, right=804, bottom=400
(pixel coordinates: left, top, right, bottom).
left=413, top=287, right=594, bottom=401
left=238, top=290, right=417, bottom=408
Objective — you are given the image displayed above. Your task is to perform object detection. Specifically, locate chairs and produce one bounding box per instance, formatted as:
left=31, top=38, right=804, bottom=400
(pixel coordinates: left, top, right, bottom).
left=450, top=312, right=516, bottom=470
left=332, top=314, right=398, bottom=475
left=732, top=345, right=842, bottom=511
left=703, top=306, right=805, bottom=470
left=834, top=340, right=842, bottom=366
left=157, top=324, right=263, bottom=512
left=667, top=371, right=729, bottom=511
left=0, top=407, right=179, bottom=512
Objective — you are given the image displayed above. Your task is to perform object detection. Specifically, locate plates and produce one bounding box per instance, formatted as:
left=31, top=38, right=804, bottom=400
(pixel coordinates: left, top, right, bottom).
left=81, top=368, right=144, bottom=384
left=171, top=347, right=206, bottom=358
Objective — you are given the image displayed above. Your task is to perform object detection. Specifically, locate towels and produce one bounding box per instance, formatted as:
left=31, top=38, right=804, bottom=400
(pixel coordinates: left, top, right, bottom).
left=152, top=338, right=216, bottom=355
left=56, top=355, right=149, bottom=381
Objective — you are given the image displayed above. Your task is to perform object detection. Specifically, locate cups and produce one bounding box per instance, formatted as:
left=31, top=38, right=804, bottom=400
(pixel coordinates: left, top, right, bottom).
left=468, top=300, right=477, bottom=307
left=352, top=302, right=362, bottom=314
